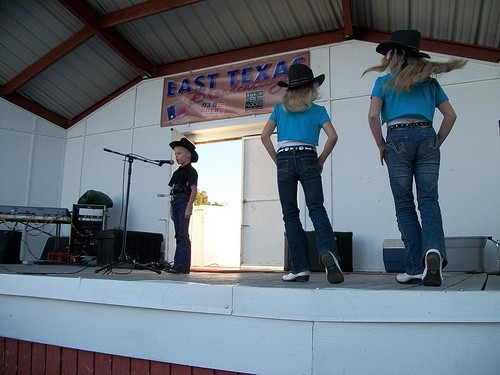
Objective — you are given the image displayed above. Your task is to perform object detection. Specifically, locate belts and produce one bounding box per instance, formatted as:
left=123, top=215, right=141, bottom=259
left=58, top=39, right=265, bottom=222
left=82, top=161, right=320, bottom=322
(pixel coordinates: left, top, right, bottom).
left=387, top=122, right=430, bottom=130
left=277, top=147, right=316, bottom=154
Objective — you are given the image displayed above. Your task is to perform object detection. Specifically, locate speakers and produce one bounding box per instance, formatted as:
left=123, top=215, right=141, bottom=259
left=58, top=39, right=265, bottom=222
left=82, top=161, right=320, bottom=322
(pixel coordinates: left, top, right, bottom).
left=68, top=204, right=105, bottom=264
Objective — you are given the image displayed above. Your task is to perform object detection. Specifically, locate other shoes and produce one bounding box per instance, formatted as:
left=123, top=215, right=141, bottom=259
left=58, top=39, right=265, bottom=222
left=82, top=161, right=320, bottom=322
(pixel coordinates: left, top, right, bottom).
left=166, top=268, right=190, bottom=274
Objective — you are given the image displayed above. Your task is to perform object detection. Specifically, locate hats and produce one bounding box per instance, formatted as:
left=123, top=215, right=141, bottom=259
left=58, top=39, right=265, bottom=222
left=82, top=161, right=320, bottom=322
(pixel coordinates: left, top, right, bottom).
left=375, top=30, right=431, bottom=60
left=168, top=137, right=199, bottom=163
left=276, top=63, right=325, bottom=89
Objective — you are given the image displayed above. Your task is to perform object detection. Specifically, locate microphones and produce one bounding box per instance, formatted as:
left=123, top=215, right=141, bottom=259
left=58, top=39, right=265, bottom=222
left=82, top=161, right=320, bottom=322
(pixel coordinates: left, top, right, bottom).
left=155, top=159, right=174, bottom=165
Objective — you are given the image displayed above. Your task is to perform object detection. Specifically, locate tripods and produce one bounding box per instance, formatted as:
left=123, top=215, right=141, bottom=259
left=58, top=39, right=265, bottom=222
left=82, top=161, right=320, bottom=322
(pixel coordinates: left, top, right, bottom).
left=94, top=147, right=163, bottom=275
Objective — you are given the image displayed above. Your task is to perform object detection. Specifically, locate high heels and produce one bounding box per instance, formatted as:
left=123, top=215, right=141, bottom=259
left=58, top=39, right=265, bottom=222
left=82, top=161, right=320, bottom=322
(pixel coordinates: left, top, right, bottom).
left=422, top=249, right=443, bottom=287
left=282, top=270, right=310, bottom=282
left=321, top=251, right=344, bottom=284
left=396, top=273, right=423, bottom=285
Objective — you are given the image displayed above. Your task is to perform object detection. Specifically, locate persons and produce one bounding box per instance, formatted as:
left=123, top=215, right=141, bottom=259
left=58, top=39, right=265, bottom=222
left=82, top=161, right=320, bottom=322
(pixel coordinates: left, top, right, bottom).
left=261, top=63, right=344, bottom=284
left=168, top=138, right=199, bottom=273
left=364, top=30, right=466, bottom=287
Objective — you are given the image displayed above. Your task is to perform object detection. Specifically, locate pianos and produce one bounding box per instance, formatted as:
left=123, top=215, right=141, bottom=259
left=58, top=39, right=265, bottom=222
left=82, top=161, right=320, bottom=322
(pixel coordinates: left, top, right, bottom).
left=0, top=205, right=72, bottom=265
